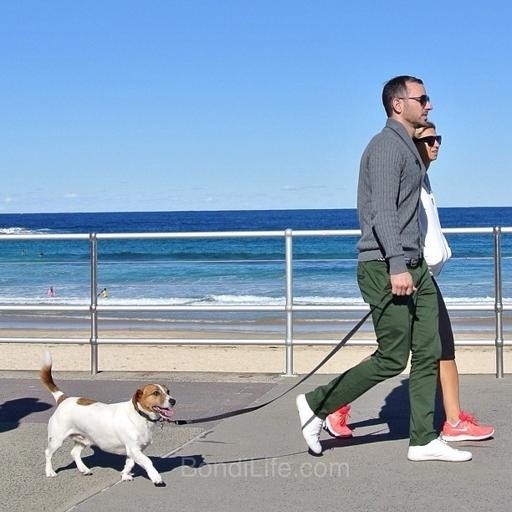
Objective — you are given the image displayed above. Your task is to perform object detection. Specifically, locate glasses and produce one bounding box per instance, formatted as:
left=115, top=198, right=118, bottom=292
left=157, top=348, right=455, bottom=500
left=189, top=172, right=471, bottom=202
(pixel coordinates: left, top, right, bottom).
left=397, top=93, right=430, bottom=107
left=415, top=135, right=442, bottom=147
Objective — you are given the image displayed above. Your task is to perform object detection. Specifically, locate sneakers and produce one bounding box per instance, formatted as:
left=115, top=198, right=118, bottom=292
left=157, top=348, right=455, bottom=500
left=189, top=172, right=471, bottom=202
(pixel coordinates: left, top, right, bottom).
left=323, top=404, right=355, bottom=439
left=294, top=392, right=324, bottom=455
left=406, top=437, right=475, bottom=464
left=440, top=411, right=496, bottom=444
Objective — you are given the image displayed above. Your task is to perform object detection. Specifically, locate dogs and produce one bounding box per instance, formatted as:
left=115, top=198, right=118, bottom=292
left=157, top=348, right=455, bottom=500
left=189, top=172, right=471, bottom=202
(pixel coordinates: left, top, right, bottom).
left=40, top=351, right=176, bottom=485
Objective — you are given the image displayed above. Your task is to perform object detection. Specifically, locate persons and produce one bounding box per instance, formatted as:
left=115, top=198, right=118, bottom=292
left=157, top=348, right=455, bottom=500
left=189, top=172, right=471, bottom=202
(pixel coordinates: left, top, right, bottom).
left=48, top=287, right=54, bottom=297
left=100, top=288, right=108, bottom=298
left=324, top=122, right=494, bottom=440
left=296, top=76, right=472, bottom=462
